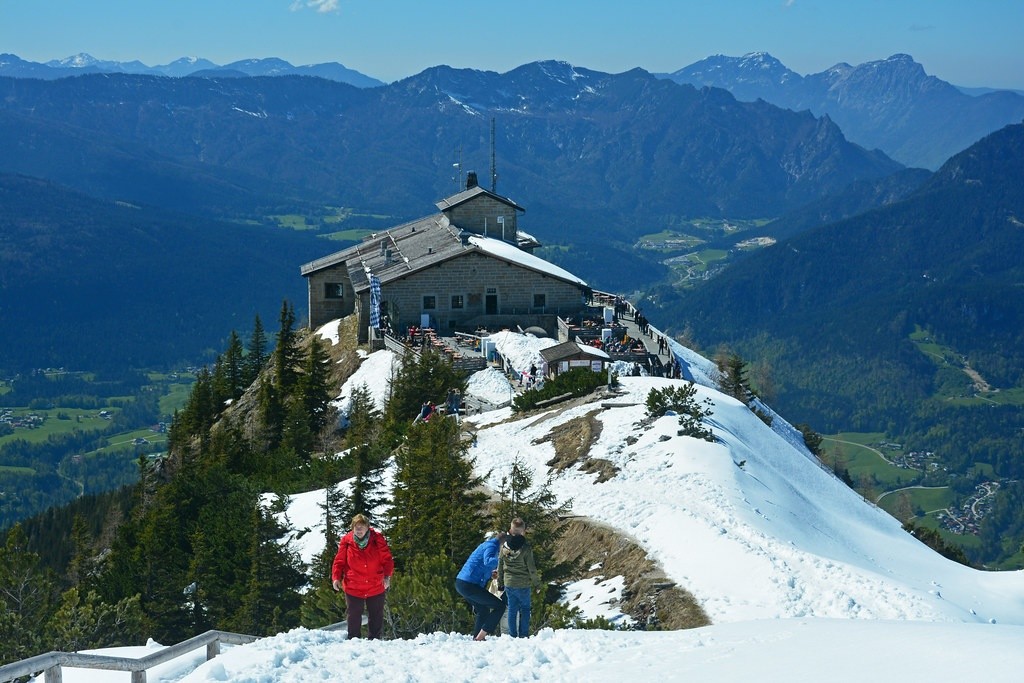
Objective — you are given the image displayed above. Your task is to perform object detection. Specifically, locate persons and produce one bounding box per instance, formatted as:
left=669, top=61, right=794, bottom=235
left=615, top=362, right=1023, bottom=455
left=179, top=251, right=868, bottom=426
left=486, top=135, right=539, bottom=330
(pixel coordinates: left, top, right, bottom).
left=452, top=530, right=506, bottom=641
left=497, top=517, right=540, bottom=640
left=485, top=570, right=527, bottom=637
left=332, top=514, right=394, bottom=642
left=387, top=287, right=683, bottom=426
